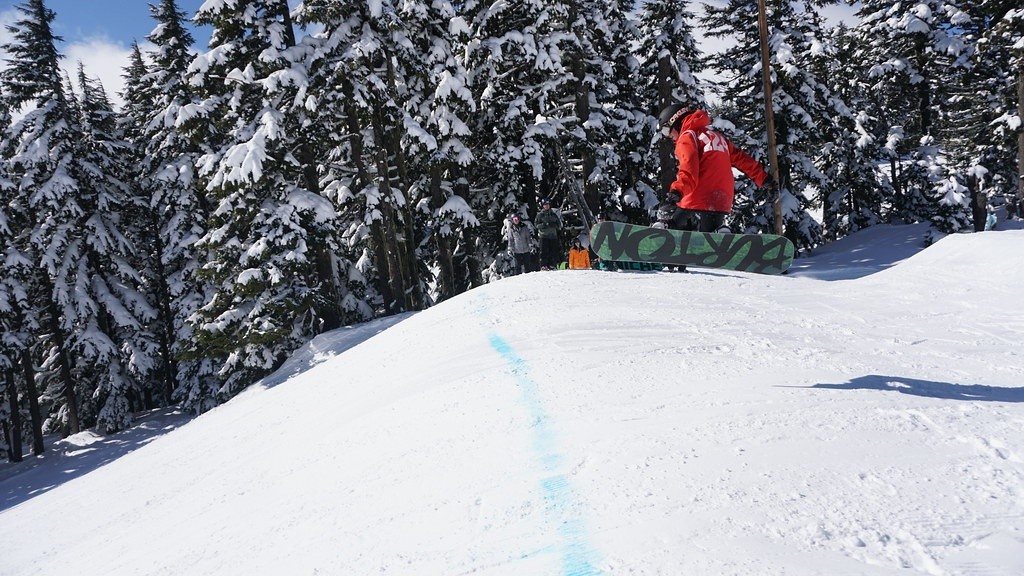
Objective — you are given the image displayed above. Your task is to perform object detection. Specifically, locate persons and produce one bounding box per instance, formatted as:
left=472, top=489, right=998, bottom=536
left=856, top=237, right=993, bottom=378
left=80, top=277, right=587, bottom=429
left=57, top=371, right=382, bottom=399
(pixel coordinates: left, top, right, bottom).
left=569, top=237, right=591, bottom=269
left=508, top=199, right=561, bottom=275
left=659, top=104, right=779, bottom=232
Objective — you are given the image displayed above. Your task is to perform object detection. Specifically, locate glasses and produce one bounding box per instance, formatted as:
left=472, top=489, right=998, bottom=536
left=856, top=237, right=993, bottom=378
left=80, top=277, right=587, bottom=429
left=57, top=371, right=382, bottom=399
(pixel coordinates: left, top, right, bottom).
left=661, top=122, right=672, bottom=136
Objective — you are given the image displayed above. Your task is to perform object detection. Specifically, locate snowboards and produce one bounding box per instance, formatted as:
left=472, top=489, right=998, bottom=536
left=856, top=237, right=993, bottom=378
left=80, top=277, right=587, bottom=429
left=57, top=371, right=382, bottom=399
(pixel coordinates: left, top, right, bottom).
left=589, top=220, right=795, bottom=276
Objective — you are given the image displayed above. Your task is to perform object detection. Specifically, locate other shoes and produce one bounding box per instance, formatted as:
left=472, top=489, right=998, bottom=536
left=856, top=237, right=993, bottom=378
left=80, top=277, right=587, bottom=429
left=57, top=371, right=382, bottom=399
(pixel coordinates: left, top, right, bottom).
left=617, top=268, right=623, bottom=273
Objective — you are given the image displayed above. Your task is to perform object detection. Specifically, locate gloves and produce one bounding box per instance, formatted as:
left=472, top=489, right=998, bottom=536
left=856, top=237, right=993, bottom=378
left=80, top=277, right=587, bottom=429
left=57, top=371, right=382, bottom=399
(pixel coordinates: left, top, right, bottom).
left=657, top=190, right=681, bottom=221
left=545, top=222, right=556, bottom=226
left=507, top=247, right=515, bottom=256
left=762, top=175, right=778, bottom=196
left=531, top=245, right=537, bottom=253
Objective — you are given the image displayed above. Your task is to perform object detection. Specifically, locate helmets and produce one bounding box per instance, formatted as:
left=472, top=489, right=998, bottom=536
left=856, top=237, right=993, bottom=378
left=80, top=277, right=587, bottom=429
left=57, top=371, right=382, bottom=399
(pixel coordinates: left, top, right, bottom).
left=656, top=104, right=690, bottom=132
left=511, top=214, right=519, bottom=220
left=542, top=198, right=551, bottom=205
